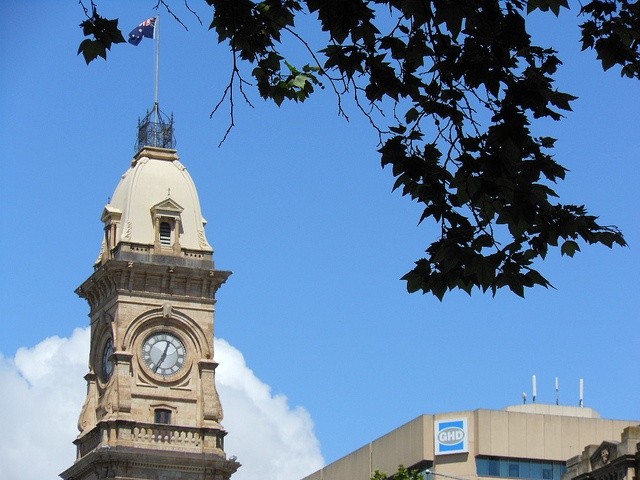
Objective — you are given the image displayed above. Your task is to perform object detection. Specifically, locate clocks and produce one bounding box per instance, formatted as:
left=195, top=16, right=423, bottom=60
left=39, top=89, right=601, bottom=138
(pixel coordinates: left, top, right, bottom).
left=137, top=326, right=192, bottom=383
left=97, top=333, right=114, bottom=389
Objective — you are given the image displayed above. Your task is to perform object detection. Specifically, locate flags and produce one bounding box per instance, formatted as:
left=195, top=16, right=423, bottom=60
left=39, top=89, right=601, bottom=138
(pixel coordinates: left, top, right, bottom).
left=127, top=15, right=160, bottom=46
